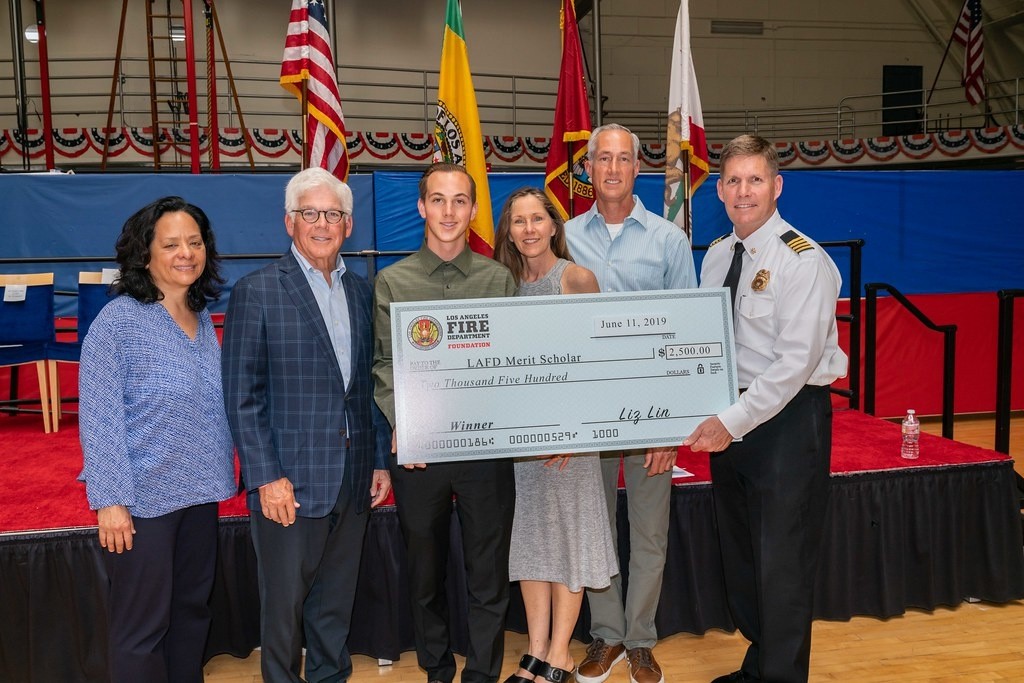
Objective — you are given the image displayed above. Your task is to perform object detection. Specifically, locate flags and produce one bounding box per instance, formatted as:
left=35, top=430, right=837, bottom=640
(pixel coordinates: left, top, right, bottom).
left=432, top=0, right=496, bottom=259
left=279, top=0, right=350, bottom=183
left=544, top=0, right=593, bottom=223
left=953, top=0, right=986, bottom=106
left=663, top=0, right=709, bottom=251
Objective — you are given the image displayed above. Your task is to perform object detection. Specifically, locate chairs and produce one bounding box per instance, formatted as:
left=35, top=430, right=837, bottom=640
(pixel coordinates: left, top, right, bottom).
left=0, top=273, right=63, bottom=436
left=45, top=269, right=123, bottom=433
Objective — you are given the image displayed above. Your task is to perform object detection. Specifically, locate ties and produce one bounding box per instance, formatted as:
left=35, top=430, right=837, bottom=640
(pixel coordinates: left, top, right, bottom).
left=722, top=243, right=745, bottom=324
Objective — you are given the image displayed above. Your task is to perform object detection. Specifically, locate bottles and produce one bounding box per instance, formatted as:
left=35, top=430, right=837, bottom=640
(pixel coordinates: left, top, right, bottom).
left=901, top=409, right=921, bottom=459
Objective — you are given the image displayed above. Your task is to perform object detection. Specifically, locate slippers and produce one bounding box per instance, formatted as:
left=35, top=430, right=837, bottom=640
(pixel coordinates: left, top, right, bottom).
left=502, top=654, right=576, bottom=683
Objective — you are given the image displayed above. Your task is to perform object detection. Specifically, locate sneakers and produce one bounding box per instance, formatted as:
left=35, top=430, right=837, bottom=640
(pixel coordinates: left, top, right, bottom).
left=625, top=648, right=664, bottom=683
left=576, top=638, right=625, bottom=683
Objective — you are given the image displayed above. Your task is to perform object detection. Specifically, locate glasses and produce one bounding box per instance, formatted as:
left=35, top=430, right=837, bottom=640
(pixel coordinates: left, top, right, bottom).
left=290, top=208, right=347, bottom=224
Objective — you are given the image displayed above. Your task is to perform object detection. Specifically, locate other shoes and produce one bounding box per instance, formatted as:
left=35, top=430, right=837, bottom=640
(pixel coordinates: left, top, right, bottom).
left=711, top=670, right=746, bottom=683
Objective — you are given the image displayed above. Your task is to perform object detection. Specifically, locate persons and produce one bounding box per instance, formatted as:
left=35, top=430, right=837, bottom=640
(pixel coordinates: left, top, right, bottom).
left=371, top=163, right=517, bottom=683
left=493, top=186, right=620, bottom=683
left=564, top=124, right=698, bottom=683
left=76, top=195, right=239, bottom=683
left=683, top=136, right=848, bottom=682
left=221, top=166, right=390, bottom=683
